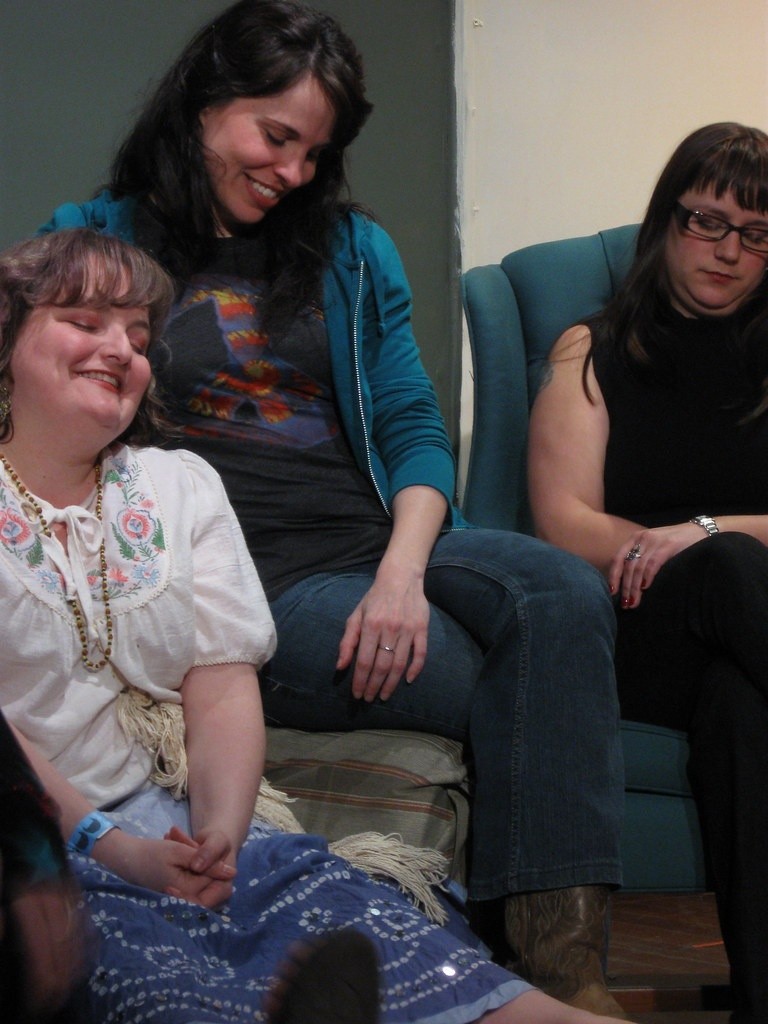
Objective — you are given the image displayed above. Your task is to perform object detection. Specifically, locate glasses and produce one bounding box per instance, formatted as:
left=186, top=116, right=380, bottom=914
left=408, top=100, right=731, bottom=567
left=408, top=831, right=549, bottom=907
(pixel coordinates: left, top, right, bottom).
left=671, top=198, right=768, bottom=254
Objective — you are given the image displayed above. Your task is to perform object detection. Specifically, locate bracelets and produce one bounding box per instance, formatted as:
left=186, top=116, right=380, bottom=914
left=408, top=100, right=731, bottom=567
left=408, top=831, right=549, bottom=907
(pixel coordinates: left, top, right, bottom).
left=66, top=809, right=121, bottom=857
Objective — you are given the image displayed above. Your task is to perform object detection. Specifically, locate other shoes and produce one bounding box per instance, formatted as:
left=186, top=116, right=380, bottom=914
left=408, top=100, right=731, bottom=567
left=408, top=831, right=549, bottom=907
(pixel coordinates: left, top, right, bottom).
left=263, top=928, right=382, bottom=1024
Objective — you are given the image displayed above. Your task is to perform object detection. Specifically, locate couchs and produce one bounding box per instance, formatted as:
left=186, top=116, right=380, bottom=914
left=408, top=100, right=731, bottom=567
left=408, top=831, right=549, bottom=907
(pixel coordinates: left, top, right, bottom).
left=463, top=224, right=707, bottom=894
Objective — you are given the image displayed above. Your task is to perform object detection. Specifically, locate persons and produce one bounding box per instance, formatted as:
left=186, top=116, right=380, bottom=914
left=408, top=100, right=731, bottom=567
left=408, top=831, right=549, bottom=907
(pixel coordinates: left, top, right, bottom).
left=525, top=117, right=768, bottom=982
left=0, top=228, right=647, bottom=1024
left=24, top=1, right=628, bottom=1024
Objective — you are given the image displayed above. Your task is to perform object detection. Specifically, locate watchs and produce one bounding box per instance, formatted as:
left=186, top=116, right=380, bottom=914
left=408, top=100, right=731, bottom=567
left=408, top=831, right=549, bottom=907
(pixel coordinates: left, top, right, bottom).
left=690, top=514, right=720, bottom=539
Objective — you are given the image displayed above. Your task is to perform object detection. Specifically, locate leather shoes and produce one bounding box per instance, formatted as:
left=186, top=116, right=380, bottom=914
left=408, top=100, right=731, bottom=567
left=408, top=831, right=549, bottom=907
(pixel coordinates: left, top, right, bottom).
left=502, top=883, right=634, bottom=1022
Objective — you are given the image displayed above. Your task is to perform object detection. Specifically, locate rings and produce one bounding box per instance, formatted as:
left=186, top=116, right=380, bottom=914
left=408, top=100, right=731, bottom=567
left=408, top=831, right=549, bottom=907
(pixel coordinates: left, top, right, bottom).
left=378, top=645, right=395, bottom=655
left=625, top=544, right=644, bottom=562
